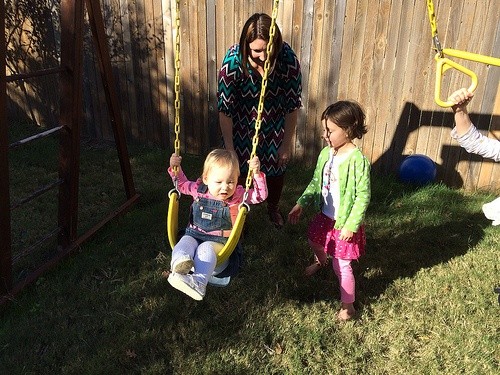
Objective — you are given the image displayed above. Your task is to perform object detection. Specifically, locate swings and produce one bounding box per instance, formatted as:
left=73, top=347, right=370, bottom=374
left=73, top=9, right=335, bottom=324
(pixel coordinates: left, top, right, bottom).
left=167, top=0, right=279, bottom=265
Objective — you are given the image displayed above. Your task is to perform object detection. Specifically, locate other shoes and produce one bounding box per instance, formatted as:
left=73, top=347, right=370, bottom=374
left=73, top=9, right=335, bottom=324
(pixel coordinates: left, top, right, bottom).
left=242, top=221, right=250, bottom=238
left=266, top=203, right=284, bottom=229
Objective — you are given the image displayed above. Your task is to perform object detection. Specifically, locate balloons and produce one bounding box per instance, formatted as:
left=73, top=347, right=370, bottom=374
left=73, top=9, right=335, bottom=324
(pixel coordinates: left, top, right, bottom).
left=399, top=155, right=436, bottom=186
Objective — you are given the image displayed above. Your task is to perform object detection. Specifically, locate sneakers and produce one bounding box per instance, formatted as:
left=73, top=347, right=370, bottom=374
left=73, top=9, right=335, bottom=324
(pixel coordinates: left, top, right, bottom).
left=167, top=272, right=207, bottom=300
left=172, top=255, right=194, bottom=276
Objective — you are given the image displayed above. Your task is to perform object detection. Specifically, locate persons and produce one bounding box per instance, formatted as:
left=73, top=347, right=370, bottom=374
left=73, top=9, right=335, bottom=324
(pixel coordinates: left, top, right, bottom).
left=448, top=88, right=500, bottom=227
left=288, top=100, right=371, bottom=322
left=169, top=149, right=267, bottom=301
left=217, top=14, right=303, bottom=230
